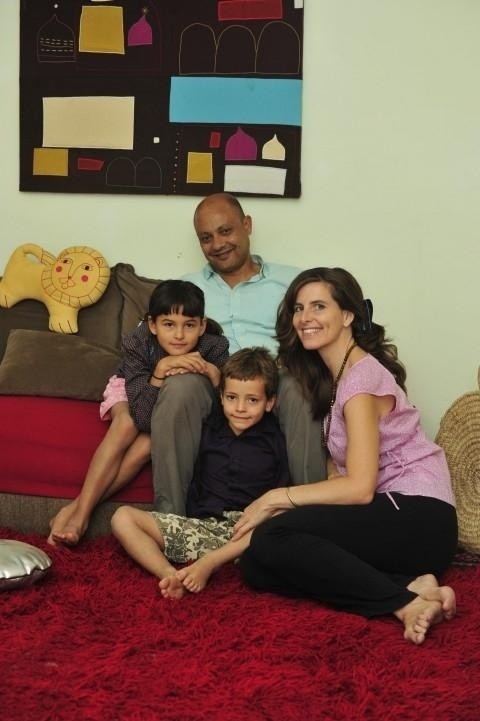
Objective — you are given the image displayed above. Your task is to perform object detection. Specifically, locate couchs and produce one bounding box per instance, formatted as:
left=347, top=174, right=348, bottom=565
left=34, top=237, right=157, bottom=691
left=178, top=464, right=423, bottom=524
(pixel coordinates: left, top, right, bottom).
left=0, top=262, right=155, bottom=544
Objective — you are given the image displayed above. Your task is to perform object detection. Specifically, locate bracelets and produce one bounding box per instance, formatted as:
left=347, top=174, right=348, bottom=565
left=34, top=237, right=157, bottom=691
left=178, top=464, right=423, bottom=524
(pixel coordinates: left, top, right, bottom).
left=286, top=487, right=297, bottom=508
left=152, top=374, right=165, bottom=380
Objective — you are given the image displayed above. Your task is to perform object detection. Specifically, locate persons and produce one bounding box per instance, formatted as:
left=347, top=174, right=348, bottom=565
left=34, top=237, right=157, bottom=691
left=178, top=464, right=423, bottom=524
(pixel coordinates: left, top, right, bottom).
left=47, top=280, right=230, bottom=547
left=111, top=346, right=290, bottom=602
left=232, top=266, right=459, bottom=643
left=139, top=193, right=329, bottom=488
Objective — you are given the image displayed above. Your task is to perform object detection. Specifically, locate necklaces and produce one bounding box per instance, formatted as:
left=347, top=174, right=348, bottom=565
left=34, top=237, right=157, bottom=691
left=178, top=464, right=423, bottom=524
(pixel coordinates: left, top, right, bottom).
left=320, top=342, right=359, bottom=448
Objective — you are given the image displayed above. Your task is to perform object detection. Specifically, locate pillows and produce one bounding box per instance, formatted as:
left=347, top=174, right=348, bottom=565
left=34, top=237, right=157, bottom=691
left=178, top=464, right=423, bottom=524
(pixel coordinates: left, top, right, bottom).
left=0, top=327, right=124, bottom=400
